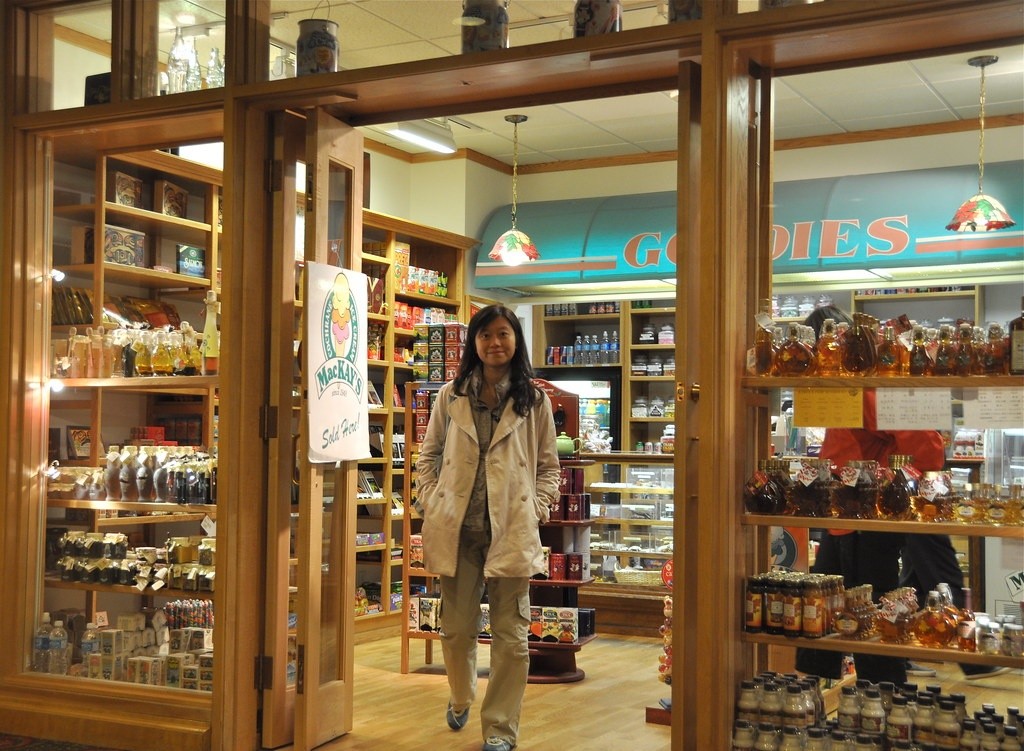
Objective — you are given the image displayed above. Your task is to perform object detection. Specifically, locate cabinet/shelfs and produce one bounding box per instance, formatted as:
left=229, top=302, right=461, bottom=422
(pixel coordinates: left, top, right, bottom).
left=735, top=45, right=1024, bottom=751
left=554, top=446, right=990, bottom=668
left=403, top=375, right=594, bottom=682
left=48, top=150, right=480, bottom=662
left=534, top=283, right=986, bottom=566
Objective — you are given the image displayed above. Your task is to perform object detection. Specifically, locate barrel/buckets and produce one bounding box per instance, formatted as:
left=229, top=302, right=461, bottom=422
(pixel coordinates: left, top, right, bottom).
left=573, top=0, right=624, bottom=38
left=461, top=0, right=512, bottom=56
left=295, top=19, right=340, bottom=78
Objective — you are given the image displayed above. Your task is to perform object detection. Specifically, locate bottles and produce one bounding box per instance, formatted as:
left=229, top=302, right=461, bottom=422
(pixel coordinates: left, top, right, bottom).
left=579, top=399, right=610, bottom=427
left=48, top=621, right=68, bottom=676
left=202, top=291, right=219, bottom=376
left=732, top=670, right=1024, bottom=751
left=743, top=454, right=1024, bottom=528
left=51, top=320, right=202, bottom=379
left=609, top=331, right=619, bottom=363
left=589, top=335, right=599, bottom=364
left=33, top=611, right=52, bottom=673
left=744, top=295, right=1024, bottom=377
left=600, top=331, right=609, bottom=364
left=573, top=335, right=589, bottom=365
left=744, top=569, right=1024, bottom=659
left=80, top=623, right=100, bottom=679
left=48, top=445, right=217, bottom=505
left=157, top=25, right=225, bottom=97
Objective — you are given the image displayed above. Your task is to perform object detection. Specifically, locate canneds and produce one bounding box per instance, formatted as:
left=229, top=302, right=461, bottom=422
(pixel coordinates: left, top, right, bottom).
left=635, top=441, right=663, bottom=454
left=58, top=530, right=217, bottom=591
left=589, top=302, right=620, bottom=313
left=547, top=346, right=574, bottom=365
left=579, top=607, right=595, bottom=637
left=545, top=466, right=590, bottom=582
left=745, top=570, right=846, bottom=637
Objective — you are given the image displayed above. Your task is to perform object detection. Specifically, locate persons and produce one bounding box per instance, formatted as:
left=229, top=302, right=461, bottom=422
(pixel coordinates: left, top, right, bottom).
left=796, top=306, right=945, bottom=689
left=417, top=304, right=563, bottom=751
left=898, top=430, right=1013, bottom=679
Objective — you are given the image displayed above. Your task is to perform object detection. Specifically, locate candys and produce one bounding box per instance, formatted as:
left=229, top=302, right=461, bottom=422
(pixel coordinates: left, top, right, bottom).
left=165, top=599, right=215, bottom=628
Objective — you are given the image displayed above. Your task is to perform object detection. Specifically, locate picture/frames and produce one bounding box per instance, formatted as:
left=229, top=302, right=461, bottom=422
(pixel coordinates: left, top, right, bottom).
left=66, top=424, right=106, bottom=459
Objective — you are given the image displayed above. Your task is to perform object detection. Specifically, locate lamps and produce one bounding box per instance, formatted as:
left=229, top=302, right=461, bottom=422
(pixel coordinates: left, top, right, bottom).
left=943, top=53, right=1018, bottom=233
left=385, top=117, right=458, bottom=154
left=487, top=115, right=540, bottom=268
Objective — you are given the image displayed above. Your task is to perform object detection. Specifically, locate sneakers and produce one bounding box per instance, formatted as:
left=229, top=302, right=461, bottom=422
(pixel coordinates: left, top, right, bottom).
left=482, top=736, right=510, bottom=751
left=446, top=701, right=469, bottom=731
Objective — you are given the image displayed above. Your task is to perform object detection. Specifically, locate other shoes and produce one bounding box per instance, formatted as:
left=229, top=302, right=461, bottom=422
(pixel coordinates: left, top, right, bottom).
left=905, top=659, right=936, bottom=676
left=966, top=666, right=1012, bottom=679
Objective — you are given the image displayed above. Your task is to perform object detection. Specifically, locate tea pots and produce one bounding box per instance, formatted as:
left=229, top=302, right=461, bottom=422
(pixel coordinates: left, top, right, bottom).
left=556, top=431, right=583, bottom=456
